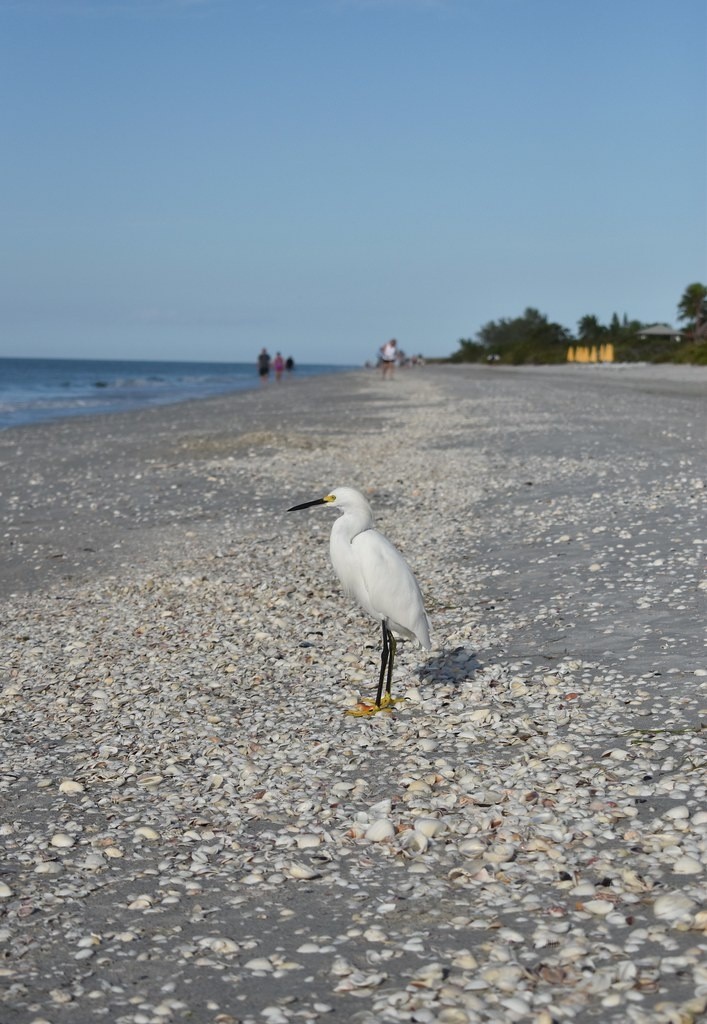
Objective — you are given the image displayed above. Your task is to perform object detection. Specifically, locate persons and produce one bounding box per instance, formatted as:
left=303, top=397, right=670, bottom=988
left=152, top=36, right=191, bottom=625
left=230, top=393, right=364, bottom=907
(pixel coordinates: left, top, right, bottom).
left=271, top=351, right=285, bottom=383
left=285, top=355, right=296, bottom=379
left=363, top=348, right=427, bottom=371
left=380, top=337, right=399, bottom=382
left=255, top=348, right=271, bottom=388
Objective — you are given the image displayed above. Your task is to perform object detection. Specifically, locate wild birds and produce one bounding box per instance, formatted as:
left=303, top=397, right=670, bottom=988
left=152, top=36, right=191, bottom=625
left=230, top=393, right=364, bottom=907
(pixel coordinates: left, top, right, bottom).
left=284, top=487, right=433, bottom=718
